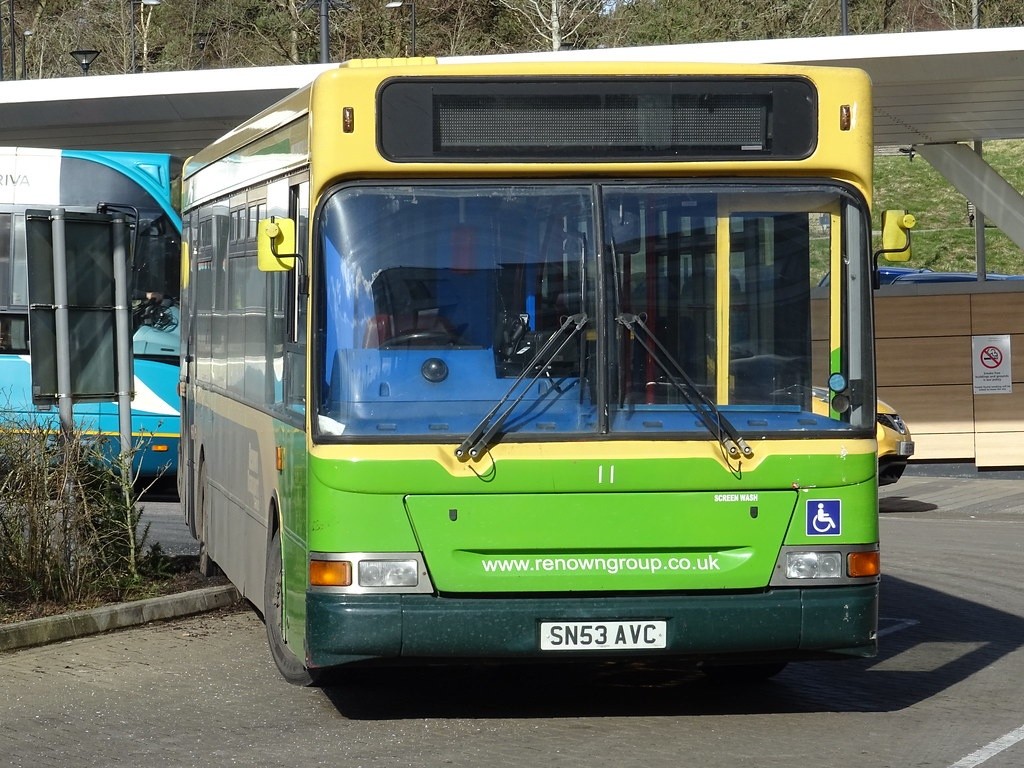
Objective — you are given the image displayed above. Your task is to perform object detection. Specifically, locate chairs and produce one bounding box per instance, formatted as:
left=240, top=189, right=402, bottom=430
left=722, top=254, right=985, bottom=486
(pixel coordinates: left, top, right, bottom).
left=363, top=314, right=456, bottom=348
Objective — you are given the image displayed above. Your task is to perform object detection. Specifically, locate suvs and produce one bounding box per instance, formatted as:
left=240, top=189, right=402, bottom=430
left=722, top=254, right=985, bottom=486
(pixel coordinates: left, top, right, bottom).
left=569, top=327, right=915, bottom=489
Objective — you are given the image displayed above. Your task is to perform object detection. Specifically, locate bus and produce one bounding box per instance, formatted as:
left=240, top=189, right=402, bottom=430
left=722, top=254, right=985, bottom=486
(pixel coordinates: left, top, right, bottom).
left=175, top=56, right=880, bottom=689
left=0, top=146, right=311, bottom=476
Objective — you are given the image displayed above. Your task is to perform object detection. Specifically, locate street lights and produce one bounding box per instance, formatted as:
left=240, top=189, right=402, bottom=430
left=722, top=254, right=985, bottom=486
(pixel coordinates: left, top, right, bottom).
left=386, top=2, right=416, bottom=56
left=129, top=0, right=160, bottom=74
left=21, top=31, right=34, bottom=79
left=70, top=49, right=101, bottom=76
